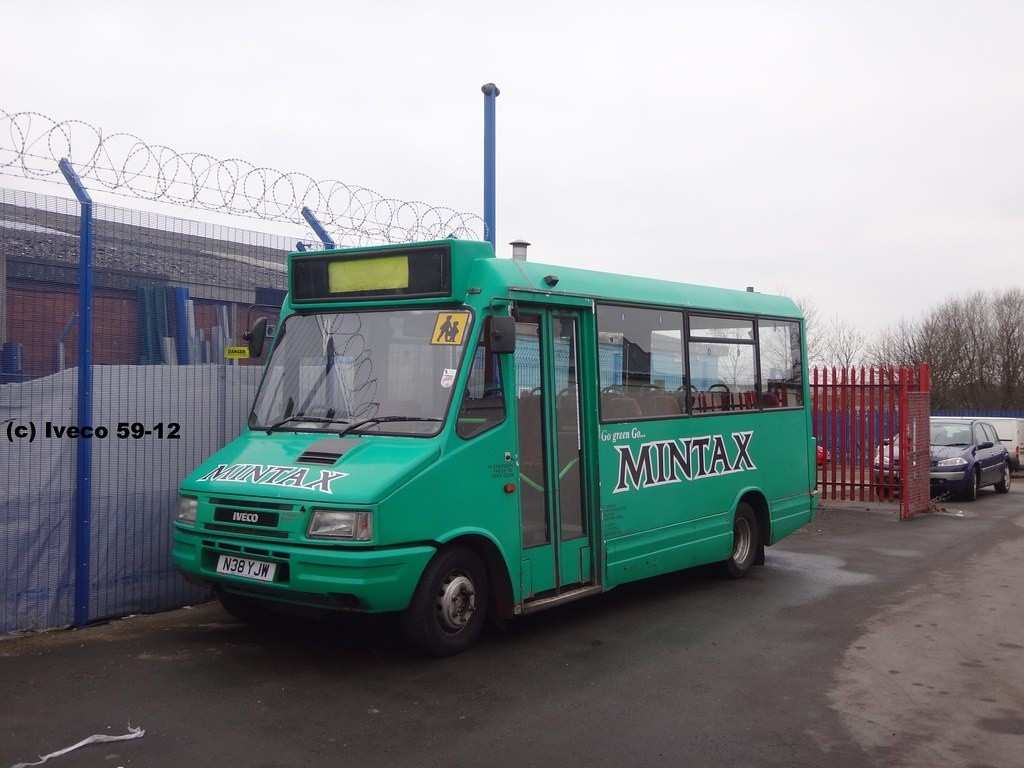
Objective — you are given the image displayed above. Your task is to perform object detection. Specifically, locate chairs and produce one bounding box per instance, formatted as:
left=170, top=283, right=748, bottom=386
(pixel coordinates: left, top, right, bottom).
left=475, top=382, right=777, bottom=448
left=934, top=426, right=973, bottom=443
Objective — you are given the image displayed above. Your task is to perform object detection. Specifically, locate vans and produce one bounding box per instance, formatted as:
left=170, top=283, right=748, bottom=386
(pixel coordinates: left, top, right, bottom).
left=876, top=416, right=1024, bottom=475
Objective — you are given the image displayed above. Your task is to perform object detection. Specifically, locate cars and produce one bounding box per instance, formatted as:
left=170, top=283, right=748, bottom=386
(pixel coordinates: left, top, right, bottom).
left=873, top=418, right=1011, bottom=501
left=817, top=446, right=830, bottom=467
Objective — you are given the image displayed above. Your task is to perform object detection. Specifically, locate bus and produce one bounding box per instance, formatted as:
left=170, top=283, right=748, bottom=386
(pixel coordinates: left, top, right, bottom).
left=168, top=232, right=820, bottom=659
